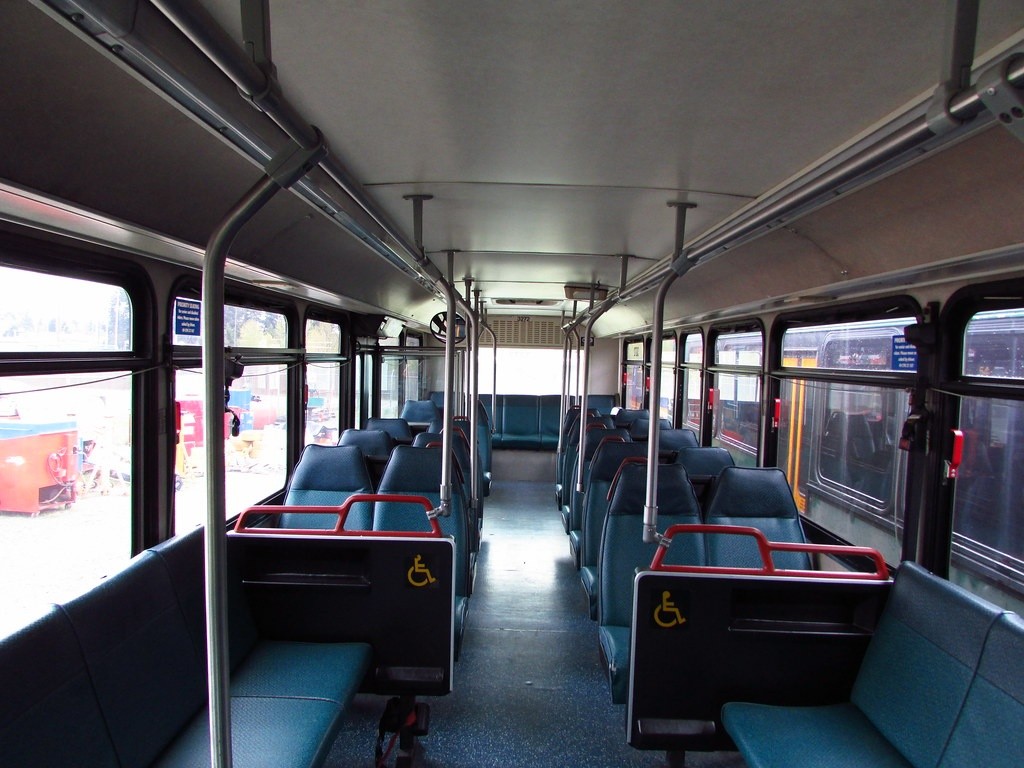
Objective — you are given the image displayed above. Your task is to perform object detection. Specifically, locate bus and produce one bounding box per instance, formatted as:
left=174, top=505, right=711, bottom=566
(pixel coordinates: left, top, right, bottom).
left=632, top=309, right=1024, bottom=566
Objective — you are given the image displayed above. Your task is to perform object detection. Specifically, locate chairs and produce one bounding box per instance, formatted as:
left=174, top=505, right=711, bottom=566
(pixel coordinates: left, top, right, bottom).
left=0, top=392, right=1024, bottom=768
